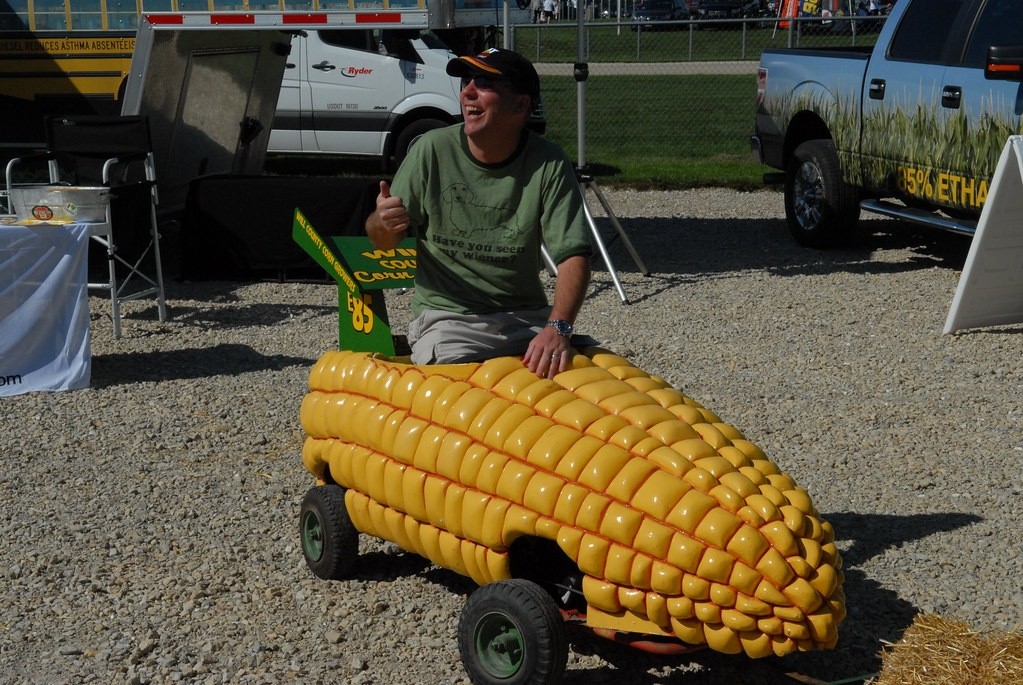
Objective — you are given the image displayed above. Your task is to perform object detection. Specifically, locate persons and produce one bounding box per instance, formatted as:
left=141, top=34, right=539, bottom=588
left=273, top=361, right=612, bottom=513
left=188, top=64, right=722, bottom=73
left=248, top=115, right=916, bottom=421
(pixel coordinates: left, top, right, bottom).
left=530, top=0, right=587, bottom=25
left=364, top=48, right=593, bottom=380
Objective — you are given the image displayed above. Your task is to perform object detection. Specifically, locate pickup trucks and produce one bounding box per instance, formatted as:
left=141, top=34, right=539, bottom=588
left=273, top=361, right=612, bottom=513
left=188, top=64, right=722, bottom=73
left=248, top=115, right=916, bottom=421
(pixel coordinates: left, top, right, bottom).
left=750, top=0, right=1023, bottom=251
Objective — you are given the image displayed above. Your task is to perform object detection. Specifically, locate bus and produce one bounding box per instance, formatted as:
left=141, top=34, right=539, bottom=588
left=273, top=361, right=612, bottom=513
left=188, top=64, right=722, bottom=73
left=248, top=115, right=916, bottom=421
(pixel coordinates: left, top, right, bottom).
left=0, top=1, right=432, bottom=121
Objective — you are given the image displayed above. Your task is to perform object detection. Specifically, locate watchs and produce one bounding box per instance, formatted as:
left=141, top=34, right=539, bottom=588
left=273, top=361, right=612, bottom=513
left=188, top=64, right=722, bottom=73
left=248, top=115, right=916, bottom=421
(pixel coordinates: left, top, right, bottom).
left=545, top=318, right=573, bottom=336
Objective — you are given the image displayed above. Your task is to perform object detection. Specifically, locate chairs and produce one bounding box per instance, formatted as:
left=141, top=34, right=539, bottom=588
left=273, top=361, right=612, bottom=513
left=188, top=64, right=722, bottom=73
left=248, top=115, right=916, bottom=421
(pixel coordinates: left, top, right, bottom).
left=6, top=114, right=167, bottom=340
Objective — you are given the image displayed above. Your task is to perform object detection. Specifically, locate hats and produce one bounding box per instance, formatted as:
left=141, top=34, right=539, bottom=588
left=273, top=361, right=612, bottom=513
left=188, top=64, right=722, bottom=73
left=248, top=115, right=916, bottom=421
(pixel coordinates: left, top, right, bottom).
left=446, top=47, right=540, bottom=98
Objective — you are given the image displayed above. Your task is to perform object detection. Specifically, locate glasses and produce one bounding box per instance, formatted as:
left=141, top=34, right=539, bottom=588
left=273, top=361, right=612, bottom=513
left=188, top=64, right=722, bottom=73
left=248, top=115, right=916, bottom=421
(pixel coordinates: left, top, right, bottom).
left=461, top=74, right=514, bottom=91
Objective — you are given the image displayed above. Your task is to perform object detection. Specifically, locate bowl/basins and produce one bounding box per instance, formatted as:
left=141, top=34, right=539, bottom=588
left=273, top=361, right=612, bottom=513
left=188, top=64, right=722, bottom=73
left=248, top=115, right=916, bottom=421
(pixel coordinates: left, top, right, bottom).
left=9, top=186, right=110, bottom=223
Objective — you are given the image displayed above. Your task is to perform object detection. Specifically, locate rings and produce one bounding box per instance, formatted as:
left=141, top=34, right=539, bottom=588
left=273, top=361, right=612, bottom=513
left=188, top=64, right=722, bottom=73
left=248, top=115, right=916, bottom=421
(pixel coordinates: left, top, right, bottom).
left=551, top=354, right=561, bottom=358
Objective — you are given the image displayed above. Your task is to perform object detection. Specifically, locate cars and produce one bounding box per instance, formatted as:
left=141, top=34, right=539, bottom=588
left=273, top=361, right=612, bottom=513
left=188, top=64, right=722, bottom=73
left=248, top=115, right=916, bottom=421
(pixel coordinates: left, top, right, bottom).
left=593, top=0, right=772, bottom=32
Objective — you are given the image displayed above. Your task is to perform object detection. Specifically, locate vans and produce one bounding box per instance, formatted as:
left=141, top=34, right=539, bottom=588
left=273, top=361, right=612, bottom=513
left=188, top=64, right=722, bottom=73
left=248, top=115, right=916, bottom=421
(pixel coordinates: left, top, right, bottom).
left=267, top=24, right=548, bottom=179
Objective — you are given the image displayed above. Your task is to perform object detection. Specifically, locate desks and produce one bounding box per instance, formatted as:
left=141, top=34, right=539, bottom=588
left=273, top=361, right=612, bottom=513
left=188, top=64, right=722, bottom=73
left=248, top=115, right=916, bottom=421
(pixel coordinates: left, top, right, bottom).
left=0, top=215, right=112, bottom=398
